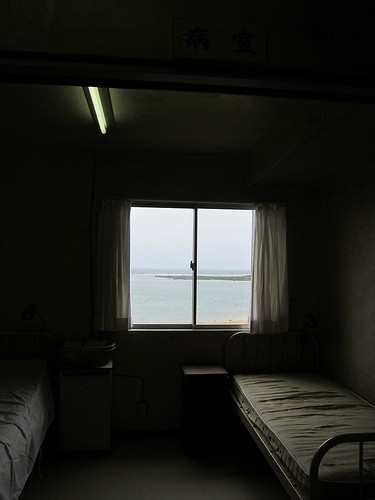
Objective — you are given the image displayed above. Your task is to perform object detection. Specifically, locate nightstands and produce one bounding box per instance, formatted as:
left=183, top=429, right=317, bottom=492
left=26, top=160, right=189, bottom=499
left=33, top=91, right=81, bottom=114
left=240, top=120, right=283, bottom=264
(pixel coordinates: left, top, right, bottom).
left=180, top=365, right=229, bottom=459
left=59, top=370, right=114, bottom=460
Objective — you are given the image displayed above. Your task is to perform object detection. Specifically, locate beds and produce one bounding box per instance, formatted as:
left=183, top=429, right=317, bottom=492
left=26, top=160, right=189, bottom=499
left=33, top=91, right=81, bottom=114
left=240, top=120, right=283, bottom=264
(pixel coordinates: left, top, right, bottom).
left=226, top=330, right=375, bottom=500
left=1, top=331, right=59, bottom=500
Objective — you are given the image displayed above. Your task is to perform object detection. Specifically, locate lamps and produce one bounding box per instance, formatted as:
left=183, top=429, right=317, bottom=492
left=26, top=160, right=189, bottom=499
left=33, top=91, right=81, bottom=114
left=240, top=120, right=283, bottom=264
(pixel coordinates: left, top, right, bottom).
left=84, top=86, right=116, bottom=137
left=19, top=304, right=52, bottom=338
